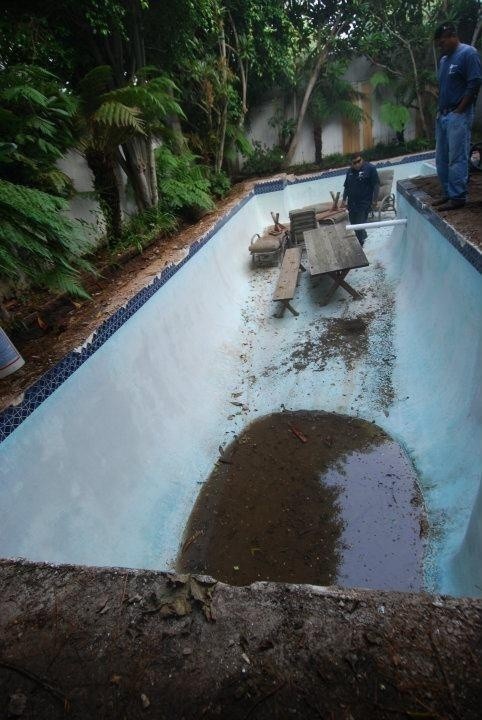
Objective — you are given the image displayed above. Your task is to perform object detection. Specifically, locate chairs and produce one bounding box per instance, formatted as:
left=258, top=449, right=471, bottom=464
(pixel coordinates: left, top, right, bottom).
left=368, top=167, right=397, bottom=221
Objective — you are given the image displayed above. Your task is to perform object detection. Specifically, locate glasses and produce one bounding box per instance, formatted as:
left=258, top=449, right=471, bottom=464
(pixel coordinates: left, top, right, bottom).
left=352, top=158, right=362, bottom=164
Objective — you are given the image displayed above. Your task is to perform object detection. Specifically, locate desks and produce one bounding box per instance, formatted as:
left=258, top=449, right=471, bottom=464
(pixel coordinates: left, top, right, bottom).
left=301, top=221, right=370, bottom=307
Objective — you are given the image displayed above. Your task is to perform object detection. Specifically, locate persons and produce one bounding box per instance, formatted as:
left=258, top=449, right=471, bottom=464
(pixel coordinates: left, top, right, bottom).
left=431, top=21, right=481, bottom=212
left=341, top=152, right=381, bottom=249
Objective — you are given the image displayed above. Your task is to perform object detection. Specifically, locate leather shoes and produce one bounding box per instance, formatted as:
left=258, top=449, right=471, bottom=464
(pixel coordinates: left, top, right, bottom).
left=431, top=197, right=466, bottom=211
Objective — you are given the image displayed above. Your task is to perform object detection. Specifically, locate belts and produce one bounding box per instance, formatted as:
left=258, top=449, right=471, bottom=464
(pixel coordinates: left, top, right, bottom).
left=440, top=105, right=456, bottom=116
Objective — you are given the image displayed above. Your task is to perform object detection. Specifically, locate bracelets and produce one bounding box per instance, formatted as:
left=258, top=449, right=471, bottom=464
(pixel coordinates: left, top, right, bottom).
left=436, top=108, right=441, bottom=114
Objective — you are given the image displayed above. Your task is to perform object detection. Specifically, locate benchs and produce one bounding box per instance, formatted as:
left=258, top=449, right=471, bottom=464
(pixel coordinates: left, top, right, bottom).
left=248, top=222, right=289, bottom=269
left=273, top=245, right=305, bottom=321
